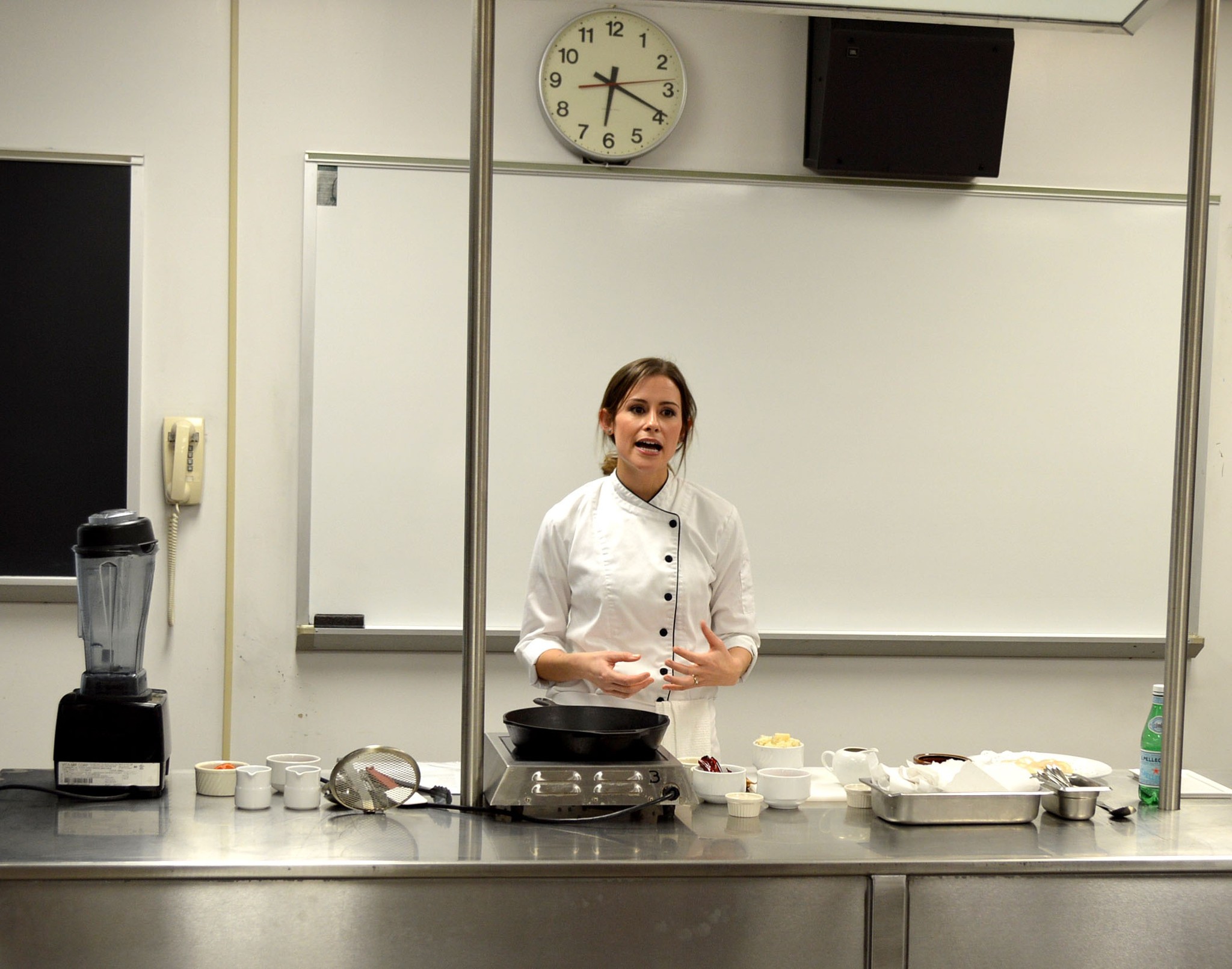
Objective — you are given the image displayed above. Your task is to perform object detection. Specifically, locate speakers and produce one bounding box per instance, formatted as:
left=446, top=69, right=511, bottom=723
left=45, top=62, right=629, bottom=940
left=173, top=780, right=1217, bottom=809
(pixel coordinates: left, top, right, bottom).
left=803, top=19, right=1015, bottom=178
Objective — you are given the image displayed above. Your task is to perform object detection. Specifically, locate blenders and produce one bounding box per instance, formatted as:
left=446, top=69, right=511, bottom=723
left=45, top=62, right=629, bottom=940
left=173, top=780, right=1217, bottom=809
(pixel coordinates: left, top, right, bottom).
left=53, top=509, right=170, bottom=797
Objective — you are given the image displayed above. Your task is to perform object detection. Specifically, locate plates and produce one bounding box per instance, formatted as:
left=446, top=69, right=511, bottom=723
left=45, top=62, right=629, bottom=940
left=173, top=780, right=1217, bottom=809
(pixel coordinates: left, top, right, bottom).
left=1128, top=768, right=1232, bottom=799
left=968, top=752, right=1112, bottom=779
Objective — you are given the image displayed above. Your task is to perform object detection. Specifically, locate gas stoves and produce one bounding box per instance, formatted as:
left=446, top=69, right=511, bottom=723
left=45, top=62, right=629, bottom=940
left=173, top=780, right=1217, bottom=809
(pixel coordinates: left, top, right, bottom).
left=483, top=732, right=700, bottom=824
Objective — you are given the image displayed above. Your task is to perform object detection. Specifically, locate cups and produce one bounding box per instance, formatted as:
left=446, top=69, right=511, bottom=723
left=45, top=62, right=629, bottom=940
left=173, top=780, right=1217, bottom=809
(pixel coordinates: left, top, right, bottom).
left=753, top=737, right=804, bottom=772
left=725, top=792, right=765, bottom=819
left=844, top=784, right=873, bottom=809
left=283, top=765, right=321, bottom=810
left=235, top=766, right=273, bottom=810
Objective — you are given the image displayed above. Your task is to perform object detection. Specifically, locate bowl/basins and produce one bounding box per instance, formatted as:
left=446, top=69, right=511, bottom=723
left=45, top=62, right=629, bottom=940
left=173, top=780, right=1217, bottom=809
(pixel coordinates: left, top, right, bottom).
left=195, top=760, right=249, bottom=796
left=267, top=754, right=321, bottom=793
left=913, top=753, right=972, bottom=765
left=676, top=757, right=811, bottom=810
left=1040, top=772, right=1113, bottom=820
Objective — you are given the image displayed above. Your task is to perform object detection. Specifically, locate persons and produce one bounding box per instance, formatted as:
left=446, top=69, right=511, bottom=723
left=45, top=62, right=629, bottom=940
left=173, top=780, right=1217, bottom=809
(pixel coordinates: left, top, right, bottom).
left=513, top=358, right=761, bottom=764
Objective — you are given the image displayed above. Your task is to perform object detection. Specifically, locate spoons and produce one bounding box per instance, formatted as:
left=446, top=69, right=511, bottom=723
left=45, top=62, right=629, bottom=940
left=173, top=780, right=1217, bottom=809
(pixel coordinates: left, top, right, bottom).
left=1096, top=801, right=1137, bottom=817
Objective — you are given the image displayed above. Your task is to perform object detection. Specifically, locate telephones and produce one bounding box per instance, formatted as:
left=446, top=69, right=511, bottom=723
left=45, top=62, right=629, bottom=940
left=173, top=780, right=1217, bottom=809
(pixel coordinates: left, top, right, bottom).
left=160, top=416, right=204, bottom=506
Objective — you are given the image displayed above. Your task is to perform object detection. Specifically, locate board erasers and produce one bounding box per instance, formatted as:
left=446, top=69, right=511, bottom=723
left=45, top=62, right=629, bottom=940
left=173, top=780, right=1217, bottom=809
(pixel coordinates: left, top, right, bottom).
left=315, top=614, right=365, bottom=628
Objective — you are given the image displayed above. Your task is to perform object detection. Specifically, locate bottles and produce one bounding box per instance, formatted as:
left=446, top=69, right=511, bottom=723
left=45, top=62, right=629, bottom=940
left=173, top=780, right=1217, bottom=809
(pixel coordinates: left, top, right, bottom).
left=1138, top=684, right=1166, bottom=805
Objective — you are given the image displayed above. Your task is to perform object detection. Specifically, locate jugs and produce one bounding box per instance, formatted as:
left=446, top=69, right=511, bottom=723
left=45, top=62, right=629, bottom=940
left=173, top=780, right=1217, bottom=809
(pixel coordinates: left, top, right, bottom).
left=821, top=746, right=880, bottom=786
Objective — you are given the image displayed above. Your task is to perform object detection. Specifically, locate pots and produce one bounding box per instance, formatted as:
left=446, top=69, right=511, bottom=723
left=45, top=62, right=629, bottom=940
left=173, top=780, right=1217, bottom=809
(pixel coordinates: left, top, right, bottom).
left=502, top=697, right=670, bottom=759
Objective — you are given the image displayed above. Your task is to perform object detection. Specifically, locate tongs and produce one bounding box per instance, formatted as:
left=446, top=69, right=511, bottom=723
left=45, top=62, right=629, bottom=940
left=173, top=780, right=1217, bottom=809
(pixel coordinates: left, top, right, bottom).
left=360, top=769, right=453, bottom=811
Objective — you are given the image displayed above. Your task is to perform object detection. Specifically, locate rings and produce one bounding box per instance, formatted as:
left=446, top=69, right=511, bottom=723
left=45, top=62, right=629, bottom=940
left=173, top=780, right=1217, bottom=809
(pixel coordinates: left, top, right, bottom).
left=692, top=675, right=699, bottom=684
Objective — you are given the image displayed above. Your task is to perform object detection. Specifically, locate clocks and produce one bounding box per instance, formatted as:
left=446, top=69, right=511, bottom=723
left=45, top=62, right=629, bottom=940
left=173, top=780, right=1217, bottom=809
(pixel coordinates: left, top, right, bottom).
left=536, top=7, right=690, bottom=162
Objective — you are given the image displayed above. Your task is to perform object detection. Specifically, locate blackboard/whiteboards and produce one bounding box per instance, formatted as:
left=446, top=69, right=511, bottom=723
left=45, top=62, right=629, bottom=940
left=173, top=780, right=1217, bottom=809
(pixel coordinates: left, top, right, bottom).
left=298, top=152, right=1222, bottom=663
